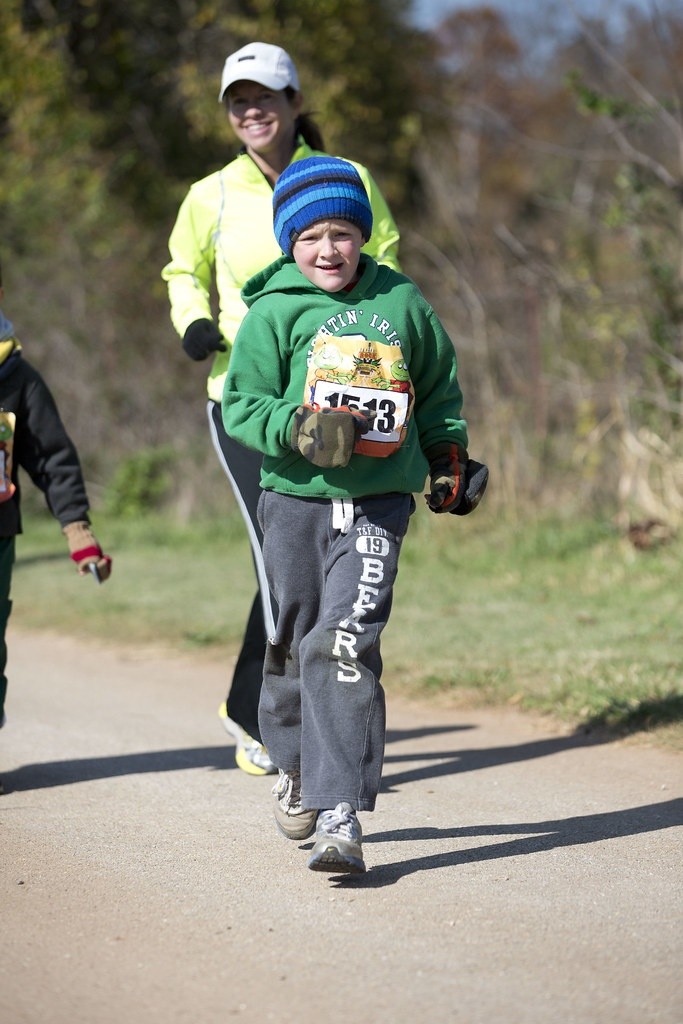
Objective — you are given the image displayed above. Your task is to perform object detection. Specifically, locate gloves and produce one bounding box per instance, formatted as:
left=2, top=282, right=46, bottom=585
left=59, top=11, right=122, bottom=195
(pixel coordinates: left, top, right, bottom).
left=425, top=441, right=489, bottom=516
left=183, top=319, right=226, bottom=361
left=65, top=522, right=111, bottom=583
left=291, top=404, right=377, bottom=470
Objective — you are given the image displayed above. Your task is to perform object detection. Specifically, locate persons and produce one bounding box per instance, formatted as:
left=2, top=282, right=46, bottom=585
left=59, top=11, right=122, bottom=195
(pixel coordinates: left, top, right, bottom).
left=162, top=42, right=400, bottom=777
left=221, top=155, right=487, bottom=872
left=1, top=312, right=112, bottom=733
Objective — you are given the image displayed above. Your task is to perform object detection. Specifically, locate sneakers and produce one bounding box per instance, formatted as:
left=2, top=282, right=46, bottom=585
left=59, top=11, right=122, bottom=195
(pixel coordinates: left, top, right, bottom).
left=307, top=801, right=366, bottom=873
left=272, top=766, right=319, bottom=839
left=218, top=702, right=279, bottom=776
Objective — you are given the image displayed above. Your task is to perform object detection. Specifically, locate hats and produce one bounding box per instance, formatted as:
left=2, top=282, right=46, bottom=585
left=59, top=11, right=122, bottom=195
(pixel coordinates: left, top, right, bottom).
left=273, top=157, right=372, bottom=259
left=218, top=41, right=299, bottom=102
